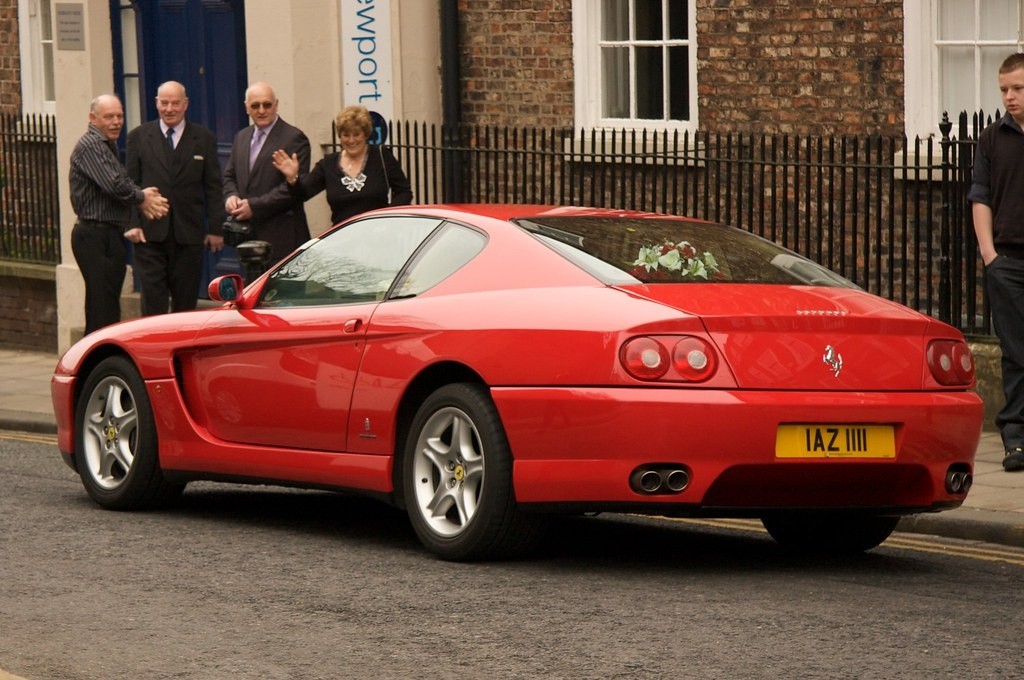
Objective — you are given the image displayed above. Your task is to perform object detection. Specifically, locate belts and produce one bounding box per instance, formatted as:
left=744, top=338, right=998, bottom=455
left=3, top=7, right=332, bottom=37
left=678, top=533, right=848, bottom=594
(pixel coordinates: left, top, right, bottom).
left=78, top=217, right=130, bottom=234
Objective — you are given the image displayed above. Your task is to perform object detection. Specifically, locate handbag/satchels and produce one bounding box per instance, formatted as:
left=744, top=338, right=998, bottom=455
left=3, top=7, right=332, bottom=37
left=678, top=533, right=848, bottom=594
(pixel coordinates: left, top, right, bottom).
left=222, top=216, right=252, bottom=249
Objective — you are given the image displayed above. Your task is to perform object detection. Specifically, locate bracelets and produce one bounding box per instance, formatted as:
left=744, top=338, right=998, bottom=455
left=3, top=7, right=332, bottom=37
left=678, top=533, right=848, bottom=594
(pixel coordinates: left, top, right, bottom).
left=286, top=175, right=298, bottom=183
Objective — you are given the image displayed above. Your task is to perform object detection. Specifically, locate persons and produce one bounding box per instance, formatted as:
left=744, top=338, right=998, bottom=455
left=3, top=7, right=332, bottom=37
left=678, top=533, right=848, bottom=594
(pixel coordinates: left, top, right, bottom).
left=220, top=83, right=310, bottom=288
left=271, top=106, right=417, bottom=229
left=965, top=52, right=1024, bottom=472
left=123, top=80, right=225, bottom=317
left=67, top=95, right=169, bottom=338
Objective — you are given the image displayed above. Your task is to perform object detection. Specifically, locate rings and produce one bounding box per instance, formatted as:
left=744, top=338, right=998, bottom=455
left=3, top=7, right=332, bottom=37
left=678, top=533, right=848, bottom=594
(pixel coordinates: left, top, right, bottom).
left=241, top=213, right=247, bottom=219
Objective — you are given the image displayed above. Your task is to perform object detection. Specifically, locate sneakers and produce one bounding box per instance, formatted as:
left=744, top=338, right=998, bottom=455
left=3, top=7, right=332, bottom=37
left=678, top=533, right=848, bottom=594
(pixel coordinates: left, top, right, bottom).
left=1002, top=447, right=1024, bottom=469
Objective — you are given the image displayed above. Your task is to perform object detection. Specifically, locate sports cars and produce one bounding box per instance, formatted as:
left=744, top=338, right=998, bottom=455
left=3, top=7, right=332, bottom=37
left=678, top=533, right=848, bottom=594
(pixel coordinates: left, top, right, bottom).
left=49, top=203, right=983, bottom=565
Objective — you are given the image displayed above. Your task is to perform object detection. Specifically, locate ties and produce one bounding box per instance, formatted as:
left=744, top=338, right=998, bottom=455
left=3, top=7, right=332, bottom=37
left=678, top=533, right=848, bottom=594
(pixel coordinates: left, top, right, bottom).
left=250, top=129, right=264, bottom=172
left=166, top=128, right=175, bottom=149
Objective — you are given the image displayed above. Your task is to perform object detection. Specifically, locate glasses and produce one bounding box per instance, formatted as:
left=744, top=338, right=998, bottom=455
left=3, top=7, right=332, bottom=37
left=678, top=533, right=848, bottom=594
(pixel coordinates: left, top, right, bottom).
left=246, top=101, right=275, bottom=110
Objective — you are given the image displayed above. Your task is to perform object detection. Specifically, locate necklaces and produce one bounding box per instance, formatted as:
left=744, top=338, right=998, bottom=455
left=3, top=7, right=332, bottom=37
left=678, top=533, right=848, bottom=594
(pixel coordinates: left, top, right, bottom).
left=348, top=162, right=353, bottom=170
